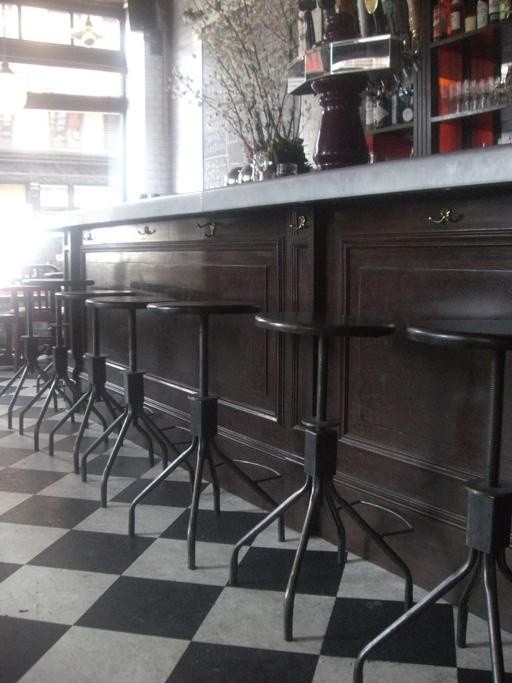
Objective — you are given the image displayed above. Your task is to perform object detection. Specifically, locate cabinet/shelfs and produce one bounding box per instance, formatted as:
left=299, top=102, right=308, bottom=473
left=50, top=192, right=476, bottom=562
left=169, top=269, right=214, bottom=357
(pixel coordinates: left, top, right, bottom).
left=355, top=0, right=512, bottom=163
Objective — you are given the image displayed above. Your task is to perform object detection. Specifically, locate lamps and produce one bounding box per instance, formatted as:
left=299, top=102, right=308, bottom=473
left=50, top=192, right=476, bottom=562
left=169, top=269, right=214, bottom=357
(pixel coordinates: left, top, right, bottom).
left=71, top=8, right=104, bottom=46
left=0, top=0, right=27, bottom=116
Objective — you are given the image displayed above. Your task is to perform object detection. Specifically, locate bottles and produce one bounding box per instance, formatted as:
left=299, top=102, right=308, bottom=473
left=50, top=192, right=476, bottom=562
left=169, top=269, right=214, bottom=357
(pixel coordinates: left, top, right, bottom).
left=365, top=83, right=413, bottom=128
left=433, top=0, right=511, bottom=41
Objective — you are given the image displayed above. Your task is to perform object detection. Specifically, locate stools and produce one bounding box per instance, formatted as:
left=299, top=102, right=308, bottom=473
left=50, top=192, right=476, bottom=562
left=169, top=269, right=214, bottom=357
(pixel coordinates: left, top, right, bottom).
left=352, top=315, right=511, bottom=683
left=228, top=309, right=414, bottom=645
left=128, top=300, right=285, bottom=570
left=0, top=264, right=156, bottom=474
left=81, top=294, right=195, bottom=509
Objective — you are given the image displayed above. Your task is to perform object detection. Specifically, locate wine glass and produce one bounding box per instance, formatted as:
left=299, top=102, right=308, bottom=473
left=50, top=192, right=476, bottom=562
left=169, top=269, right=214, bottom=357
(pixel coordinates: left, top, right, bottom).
left=447, top=77, right=501, bottom=114
left=253, top=155, right=268, bottom=180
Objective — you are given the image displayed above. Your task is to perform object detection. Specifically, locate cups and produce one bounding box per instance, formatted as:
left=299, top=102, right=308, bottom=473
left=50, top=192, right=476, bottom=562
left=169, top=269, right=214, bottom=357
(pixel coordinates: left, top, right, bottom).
left=277, top=162, right=298, bottom=177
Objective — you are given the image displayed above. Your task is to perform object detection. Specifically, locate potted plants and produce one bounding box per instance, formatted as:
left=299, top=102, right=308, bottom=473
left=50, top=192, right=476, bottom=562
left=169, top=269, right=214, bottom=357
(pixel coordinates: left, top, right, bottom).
left=193, top=0, right=311, bottom=186
